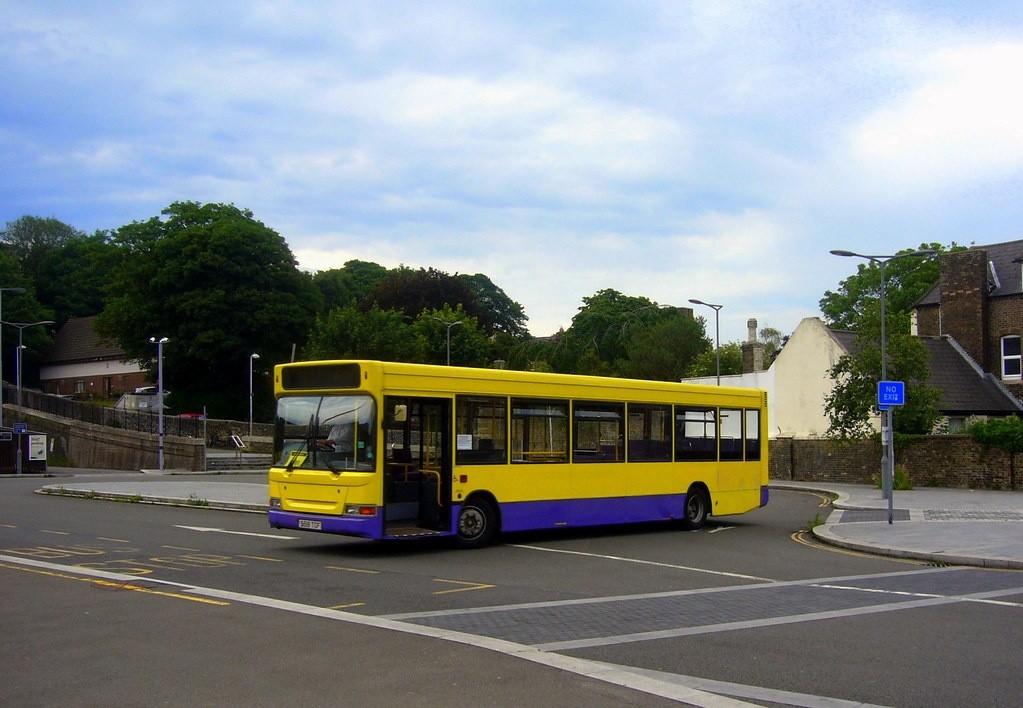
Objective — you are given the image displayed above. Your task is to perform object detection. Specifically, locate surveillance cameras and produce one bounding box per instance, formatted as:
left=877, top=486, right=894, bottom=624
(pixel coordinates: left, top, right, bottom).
left=149, top=337, right=155, bottom=343
left=161, top=338, right=170, bottom=343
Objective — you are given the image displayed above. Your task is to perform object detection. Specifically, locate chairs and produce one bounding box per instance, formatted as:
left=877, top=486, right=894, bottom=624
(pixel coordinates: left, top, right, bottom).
left=393, top=448, right=420, bottom=480
left=479, top=439, right=496, bottom=462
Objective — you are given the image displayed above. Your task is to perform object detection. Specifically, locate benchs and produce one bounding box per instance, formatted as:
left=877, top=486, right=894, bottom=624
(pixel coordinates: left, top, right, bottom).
left=572, top=450, right=606, bottom=461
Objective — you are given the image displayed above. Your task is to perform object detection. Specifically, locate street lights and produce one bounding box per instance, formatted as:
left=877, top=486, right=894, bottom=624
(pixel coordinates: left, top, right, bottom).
left=149, top=337, right=171, bottom=470
left=830, top=249, right=938, bottom=524
left=249, top=353, right=260, bottom=436
left=17, top=345, right=26, bottom=405
left=0, top=319, right=56, bottom=474
left=433, top=317, right=465, bottom=367
left=688, top=299, right=723, bottom=386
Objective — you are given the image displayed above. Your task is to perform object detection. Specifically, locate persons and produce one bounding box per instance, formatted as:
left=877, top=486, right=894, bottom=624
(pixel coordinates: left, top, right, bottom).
left=318, top=417, right=354, bottom=452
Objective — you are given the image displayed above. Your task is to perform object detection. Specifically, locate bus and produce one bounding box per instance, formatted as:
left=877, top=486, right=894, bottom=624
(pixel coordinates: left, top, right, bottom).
left=268, top=360, right=770, bottom=551
